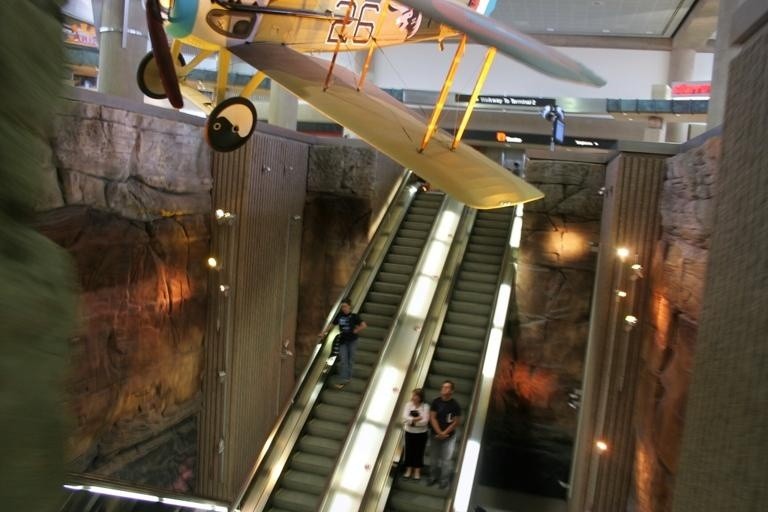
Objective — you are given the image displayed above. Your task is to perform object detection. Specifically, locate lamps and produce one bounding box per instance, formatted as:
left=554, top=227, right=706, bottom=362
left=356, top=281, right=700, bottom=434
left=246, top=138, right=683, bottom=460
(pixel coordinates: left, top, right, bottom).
left=208, top=258, right=223, bottom=272
left=598, top=187, right=613, bottom=196
left=624, top=314, right=639, bottom=332
left=282, top=339, right=293, bottom=358
left=615, top=289, right=626, bottom=302
left=220, top=284, right=230, bottom=299
left=216, top=439, right=225, bottom=455
left=620, top=251, right=628, bottom=263
left=629, top=264, right=645, bottom=281
left=216, top=369, right=226, bottom=379
left=216, top=208, right=236, bottom=225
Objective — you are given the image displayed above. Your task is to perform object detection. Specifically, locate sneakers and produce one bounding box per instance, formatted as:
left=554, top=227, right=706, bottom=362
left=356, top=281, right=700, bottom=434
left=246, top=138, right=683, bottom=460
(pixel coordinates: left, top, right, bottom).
left=426, top=477, right=449, bottom=489
left=335, top=383, right=344, bottom=390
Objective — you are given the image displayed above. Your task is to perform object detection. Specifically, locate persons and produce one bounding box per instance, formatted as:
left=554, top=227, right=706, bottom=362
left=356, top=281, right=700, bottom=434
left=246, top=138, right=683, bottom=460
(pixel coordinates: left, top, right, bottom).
left=511, top=161, right=520, bottom=177
left=425, top=380, right=461, bottom=490
left=400, top=388, right=431, bottom=481
left=317, top=298, right=367, bottom=382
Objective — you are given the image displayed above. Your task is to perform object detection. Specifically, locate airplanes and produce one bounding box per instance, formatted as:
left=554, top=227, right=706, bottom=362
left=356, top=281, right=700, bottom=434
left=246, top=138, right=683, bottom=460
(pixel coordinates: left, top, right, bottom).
left=136, top=0, right=606, bottom=210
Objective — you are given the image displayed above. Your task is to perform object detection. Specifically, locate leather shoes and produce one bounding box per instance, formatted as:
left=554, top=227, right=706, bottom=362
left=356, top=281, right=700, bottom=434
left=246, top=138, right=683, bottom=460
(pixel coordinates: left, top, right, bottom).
left=403, top=472, right=421, bottom=482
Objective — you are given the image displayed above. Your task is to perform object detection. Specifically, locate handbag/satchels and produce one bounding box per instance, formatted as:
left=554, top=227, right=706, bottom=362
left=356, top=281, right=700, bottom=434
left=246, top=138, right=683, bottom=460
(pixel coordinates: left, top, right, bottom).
left=409, top=410, right=419, bottom=426
